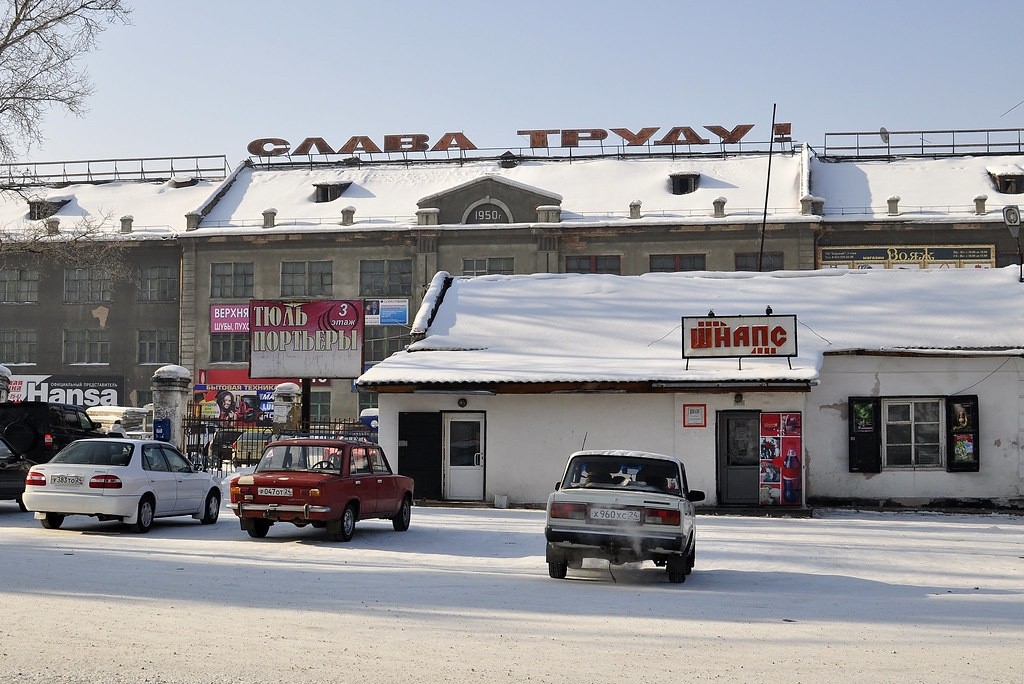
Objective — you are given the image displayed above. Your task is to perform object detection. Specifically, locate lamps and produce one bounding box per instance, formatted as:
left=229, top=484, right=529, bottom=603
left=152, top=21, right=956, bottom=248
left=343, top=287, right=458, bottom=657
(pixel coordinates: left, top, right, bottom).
left=1002, top=205, right=1024, bottom=283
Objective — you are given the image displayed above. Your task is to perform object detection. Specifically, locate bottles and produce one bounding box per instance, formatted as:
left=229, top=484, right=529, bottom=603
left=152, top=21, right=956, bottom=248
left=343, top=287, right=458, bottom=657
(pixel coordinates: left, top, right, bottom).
left=783, top=442, right=801, bottom=504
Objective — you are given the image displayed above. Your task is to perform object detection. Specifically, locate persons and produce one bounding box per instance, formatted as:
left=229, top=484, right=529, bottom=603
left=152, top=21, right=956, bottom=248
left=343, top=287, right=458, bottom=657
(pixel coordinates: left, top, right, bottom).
left=105, top=420, right=128, bottom=439
left=216, top=391, right=237, bottom=427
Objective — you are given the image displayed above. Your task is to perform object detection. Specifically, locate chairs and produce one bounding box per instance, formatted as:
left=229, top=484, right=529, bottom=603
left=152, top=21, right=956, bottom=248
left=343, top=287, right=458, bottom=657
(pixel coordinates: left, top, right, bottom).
left=92, top=454, right=108, bottom=464
left=110, top=454, right=125, bottom=465
left=586, top=471, right=612, bottom=484
left=636, top=476, right=669, bottom=490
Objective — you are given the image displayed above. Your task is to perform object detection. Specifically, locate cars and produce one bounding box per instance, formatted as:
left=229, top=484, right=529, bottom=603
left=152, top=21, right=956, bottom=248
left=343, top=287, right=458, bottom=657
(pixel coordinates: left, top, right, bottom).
left=311, top=422, right=369, bottom=440
left=544, top=450, right=705, bottom=586
left=232, top=430, right=276, bottom=470
left=226, top=438, right=415, bottom=543
left=22, top=436, right=223, bottom=533
left=0, top=435, right=41, bottom=512
left=127, top=432, right=152, bottom=441
left=323, top=437, right=379, bottom=467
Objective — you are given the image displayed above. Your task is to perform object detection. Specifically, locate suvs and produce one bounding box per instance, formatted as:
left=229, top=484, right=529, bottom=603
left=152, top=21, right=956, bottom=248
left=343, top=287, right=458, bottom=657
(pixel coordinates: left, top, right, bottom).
left=184, top=422, right=218, bottom=456
left=0, top=400, right=107, bottom=463
